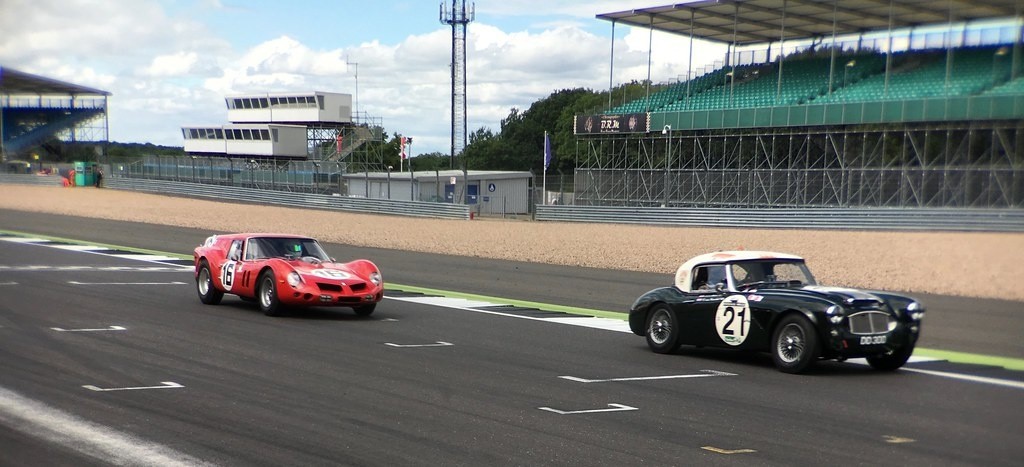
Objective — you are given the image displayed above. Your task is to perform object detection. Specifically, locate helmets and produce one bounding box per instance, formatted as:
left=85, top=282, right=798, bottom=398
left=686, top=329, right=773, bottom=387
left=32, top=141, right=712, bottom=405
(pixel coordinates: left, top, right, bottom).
left=284, top=239, right=303, bottom=260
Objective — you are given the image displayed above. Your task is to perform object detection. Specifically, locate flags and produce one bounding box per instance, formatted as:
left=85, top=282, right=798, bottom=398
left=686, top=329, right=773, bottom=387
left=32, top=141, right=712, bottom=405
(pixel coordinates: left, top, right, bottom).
left=546, top=135, right=552, bottom=170
left=336, top=136, right=343, bottom=154
left=401, top=137, right=405, bottom=159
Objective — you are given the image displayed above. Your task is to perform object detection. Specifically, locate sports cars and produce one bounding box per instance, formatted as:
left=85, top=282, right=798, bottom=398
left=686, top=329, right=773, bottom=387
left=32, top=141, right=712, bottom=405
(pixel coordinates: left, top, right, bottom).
left=628, top=251, right=927, bottom=374
left=193, top=232, right=384, bottom=318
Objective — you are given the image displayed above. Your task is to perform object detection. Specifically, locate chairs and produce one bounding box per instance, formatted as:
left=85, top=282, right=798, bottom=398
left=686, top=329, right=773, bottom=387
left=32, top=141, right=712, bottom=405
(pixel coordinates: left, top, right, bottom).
left=1, top=107, right=104, bottom=151
left=604, top=42, right=1024, bottom=113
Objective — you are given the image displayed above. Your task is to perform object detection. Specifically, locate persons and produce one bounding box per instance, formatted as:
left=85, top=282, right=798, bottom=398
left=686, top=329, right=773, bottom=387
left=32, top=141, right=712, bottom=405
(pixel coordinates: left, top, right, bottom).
left=39, top=165, right=51, bottom=175
left=698, top=269, right=738, bottom=288
left=283, top=240, right=302, bottom=258
left=61, top=168, right=75, bottom=187
left=96, top=169, right=102, bottom=188
